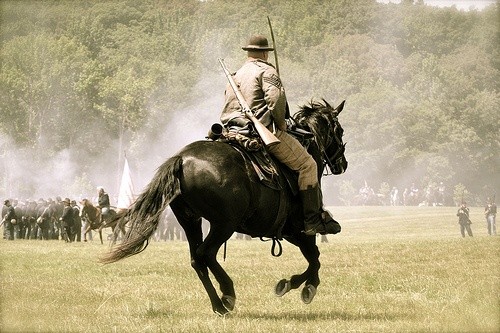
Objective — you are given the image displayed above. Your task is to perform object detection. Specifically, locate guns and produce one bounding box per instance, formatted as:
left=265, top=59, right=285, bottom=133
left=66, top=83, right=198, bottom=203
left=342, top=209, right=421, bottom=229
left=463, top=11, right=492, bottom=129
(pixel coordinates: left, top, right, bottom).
left=38, top=202, right=52, bottom=219
left=217, top=56, right=281, bottom=147
left=61, top=220, right=70, bottom=242
left=464, top=212, right=472, bottom=224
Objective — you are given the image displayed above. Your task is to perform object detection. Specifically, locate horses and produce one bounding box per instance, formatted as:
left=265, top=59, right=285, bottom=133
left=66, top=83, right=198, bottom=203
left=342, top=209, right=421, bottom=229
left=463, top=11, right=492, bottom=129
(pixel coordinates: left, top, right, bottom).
left=78, top=199, right=131, bottom=244
left=96, top=98, right=348, bottom=318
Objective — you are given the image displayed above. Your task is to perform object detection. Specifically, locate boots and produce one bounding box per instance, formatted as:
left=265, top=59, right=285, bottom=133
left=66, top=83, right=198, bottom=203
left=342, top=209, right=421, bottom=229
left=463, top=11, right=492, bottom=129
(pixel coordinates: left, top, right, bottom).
left=300, top=183, right=341, bottom=235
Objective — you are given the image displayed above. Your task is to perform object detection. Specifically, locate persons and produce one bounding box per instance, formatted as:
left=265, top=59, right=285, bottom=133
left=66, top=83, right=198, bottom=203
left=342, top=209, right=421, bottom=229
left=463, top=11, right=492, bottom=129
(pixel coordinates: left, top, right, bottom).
left=456, top=201, right=474, bottom=237
left=2, top=185, right=185, bottom=243
left=319, top=179, right=447, bottom=207
left=484, top=200, right=497, bottom=234
left=220, top=36, right=342, bottom=236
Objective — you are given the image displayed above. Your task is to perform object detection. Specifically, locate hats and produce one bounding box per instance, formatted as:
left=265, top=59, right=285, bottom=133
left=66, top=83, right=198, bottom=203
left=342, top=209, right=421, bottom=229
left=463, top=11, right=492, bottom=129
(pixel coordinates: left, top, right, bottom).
left=242, top=36, right=274, bottom=51
left=61, top=197, right=71, bottom=204
